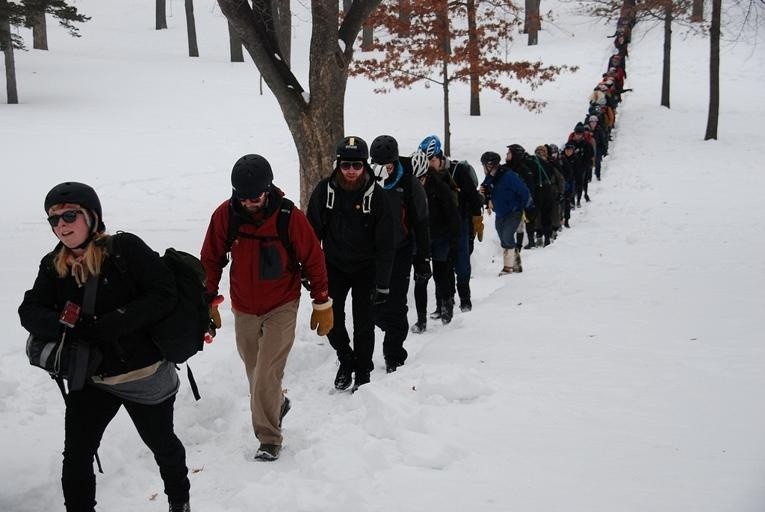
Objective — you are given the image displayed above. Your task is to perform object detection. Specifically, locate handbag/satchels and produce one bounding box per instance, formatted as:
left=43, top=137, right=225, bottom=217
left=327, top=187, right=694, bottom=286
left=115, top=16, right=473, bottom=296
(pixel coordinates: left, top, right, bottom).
left=137, top=249, right=208, bottom=366
left=25, top=329, right=101, bottom=380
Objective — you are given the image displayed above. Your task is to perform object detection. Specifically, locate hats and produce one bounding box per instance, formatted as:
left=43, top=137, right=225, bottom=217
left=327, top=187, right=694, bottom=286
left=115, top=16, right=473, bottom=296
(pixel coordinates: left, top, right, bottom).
left=535, top=146, right=546, bottom=153
left=576, top=126, right=584, bottom=134
left=589, top=115, right=598, bottom=121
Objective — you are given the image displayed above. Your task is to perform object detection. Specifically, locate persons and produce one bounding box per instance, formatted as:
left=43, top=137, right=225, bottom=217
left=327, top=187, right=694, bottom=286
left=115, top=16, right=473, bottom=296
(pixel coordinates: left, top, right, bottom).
left=200, top=153, right=336, bottom=460
left=17, top=182, right=191, bottom=512
left=307, top=137, right=484, bottom=393
left=480, top=21, right=634, bottom=276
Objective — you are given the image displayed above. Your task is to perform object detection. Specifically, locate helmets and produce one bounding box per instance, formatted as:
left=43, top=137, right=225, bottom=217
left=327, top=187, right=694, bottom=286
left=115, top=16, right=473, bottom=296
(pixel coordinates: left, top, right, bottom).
left=231, top=154, right=275, bottom=194
left=481, top=152, right=501, bottom=165
left=507, top=144, right=525, bottom=154
left=336, top=135, right=440, bottom=178
left=45, top=182, right=102, bottom=220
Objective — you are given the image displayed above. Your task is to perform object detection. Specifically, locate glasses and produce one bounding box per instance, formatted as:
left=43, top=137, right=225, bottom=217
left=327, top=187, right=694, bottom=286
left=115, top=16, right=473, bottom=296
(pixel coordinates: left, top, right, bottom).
left=239, top=195, right=262, bottom=204
left=47, top=209, right=84, bottom=227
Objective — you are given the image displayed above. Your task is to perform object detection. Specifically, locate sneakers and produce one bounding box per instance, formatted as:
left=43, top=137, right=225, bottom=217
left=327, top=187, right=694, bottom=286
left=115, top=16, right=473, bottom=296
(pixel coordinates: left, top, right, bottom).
left=524, top=223, right=570, bottom=249
left=334, top=355, right=404, bottom=394
left=571, top=194, right=591, bottom=210
left=411, top=298, right=472, bottom=333
left=499, top=266, right=523, bottom=276
left=279, top=397, right=292, bottom=427
left=254, top=444, right=282, bottom=460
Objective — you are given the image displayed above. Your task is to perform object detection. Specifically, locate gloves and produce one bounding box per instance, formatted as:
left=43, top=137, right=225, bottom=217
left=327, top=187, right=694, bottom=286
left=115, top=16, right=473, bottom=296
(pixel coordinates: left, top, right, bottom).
left=553, top=191, right=565, bottom=204
left=204, top=305, right=222, bottom=343
left=473, top=216, right=484, bottom=242
left=310, top=297, right=335, bottom=336
left=525, top=208, right=537, bottom=221
left=372, top=285, right=389, bottom=305
left=414, top=262, right=431, bottom=283
